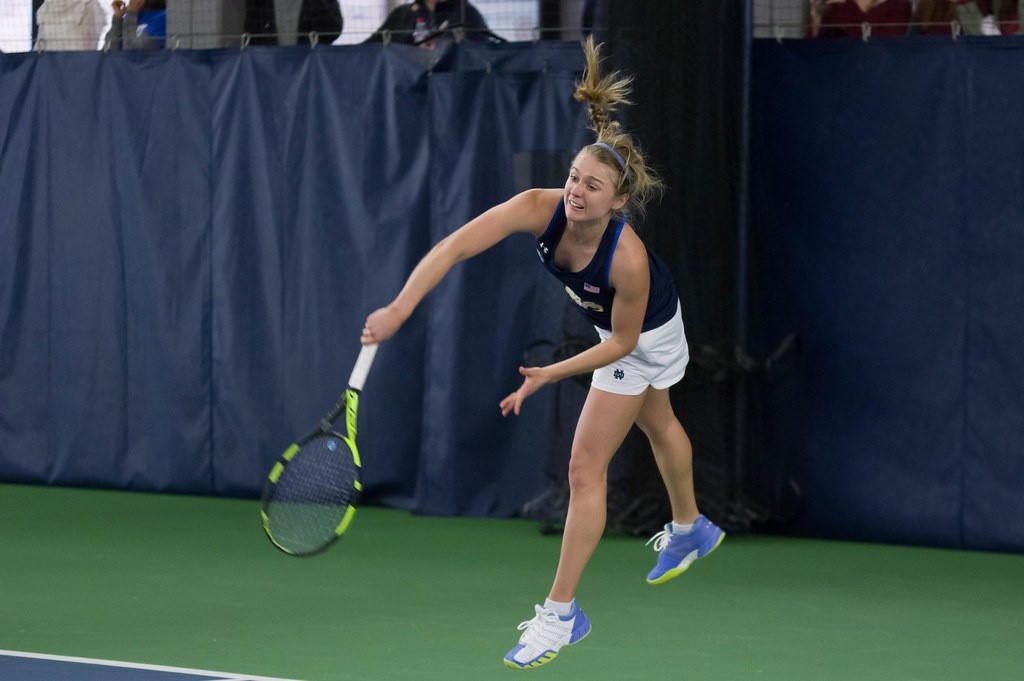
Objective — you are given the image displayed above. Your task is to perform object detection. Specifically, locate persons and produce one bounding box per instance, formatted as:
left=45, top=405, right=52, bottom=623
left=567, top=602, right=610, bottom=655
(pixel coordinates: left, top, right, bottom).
left=360, top=32, right=727, bottom=670
left=32, top=0, right=1024, bottom=50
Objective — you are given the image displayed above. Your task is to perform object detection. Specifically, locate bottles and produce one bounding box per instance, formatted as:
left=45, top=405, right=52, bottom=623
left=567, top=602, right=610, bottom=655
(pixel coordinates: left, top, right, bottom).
left=412, top=19, right=434, bottom=50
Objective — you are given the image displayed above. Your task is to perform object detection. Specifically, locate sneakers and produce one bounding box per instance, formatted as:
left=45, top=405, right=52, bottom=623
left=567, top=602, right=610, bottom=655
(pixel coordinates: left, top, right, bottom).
left=504, top=601, right=591, bottom=669
left=646, top=515, right=725, bottom=584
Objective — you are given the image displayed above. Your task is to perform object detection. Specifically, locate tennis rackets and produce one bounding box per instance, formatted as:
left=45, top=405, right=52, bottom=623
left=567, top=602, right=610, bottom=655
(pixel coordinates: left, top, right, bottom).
left=260, top=341, right=381, bottom=557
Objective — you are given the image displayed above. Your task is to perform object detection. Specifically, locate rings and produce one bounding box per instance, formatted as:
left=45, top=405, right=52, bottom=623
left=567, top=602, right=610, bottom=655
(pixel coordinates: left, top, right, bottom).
left=367, top=329, right=374, bottom=338
